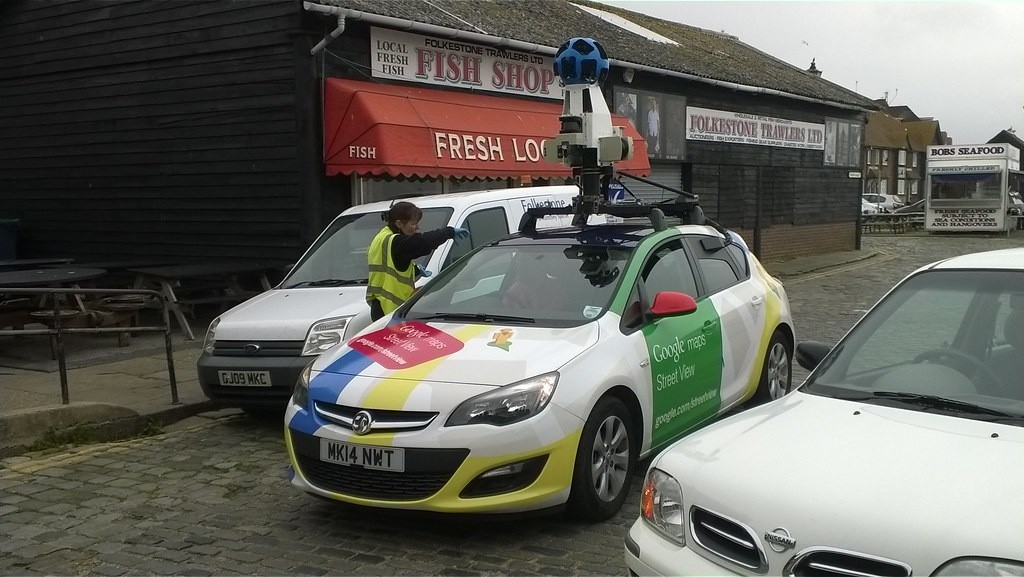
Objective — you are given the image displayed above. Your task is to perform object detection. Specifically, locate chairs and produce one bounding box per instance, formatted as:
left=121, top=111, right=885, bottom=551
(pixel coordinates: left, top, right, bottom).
left=987, top=309, right=1024, bottom=394
left=642, top=255, right=679, bottom=296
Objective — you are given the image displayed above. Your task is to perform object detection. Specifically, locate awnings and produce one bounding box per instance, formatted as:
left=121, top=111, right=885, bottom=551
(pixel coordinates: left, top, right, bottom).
left=323, top=76, right=652, bottom=181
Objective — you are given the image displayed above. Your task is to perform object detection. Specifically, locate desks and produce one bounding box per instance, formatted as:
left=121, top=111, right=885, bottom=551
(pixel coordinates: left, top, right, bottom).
left=0, top=258, right=78, bottom=268
left=0, top=267, right=131, bottom=347
left=59, top=259, right=179, bottom=329
left=127, top=260, right=285, bottom=339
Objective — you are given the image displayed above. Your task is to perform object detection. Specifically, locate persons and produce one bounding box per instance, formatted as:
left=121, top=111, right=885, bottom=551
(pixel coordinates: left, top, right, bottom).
left=647, top=98, right=660, bottom=154
left=508, top=257, right=582, bottom=313
left=365, top=201, right=471, bottom=323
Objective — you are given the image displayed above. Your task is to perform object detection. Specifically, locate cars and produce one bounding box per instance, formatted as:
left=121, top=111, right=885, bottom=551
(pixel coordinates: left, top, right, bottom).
left=285, top=203, right=1024, bottom=577
left=861, top=194, right=926, bottom=226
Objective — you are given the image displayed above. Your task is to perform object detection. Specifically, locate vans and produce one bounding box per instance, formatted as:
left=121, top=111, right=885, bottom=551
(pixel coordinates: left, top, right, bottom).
left=197, top=185, right=608, bottom=416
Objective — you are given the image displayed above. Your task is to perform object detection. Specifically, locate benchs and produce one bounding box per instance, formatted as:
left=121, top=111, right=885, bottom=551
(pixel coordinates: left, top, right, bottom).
left=1, top=282, right=262, bottom=337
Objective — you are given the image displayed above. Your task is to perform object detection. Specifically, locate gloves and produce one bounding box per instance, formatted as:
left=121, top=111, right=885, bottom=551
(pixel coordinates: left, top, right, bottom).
left=452, top=227, right=470, bottom=246
left=417, top=264, right=432, bottom=277
